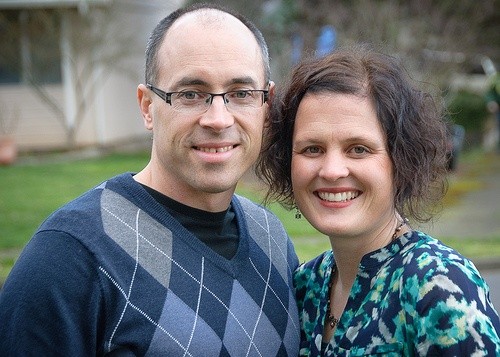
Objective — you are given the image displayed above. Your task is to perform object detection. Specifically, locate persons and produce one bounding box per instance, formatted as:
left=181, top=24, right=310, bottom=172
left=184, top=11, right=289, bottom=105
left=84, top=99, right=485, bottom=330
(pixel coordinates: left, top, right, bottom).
left=251, top=44, right=500, bottom=356
left=0, top=4, right=310, bottom=357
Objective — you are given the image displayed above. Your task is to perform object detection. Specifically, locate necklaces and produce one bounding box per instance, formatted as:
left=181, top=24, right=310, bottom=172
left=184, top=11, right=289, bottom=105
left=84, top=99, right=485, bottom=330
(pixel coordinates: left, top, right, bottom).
left=324, top=216, right=406, bottom=330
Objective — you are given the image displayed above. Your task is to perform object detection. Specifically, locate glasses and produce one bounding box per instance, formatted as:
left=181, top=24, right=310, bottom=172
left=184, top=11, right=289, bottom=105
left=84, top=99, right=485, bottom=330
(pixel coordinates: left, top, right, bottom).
left=146, top=84, right=270, bottom=112
